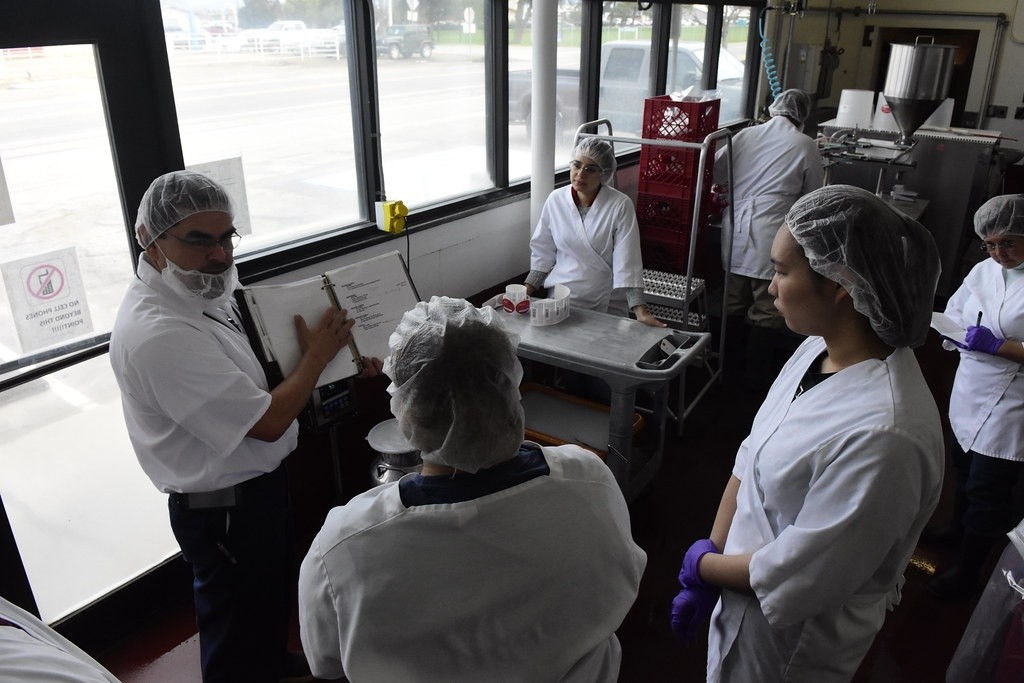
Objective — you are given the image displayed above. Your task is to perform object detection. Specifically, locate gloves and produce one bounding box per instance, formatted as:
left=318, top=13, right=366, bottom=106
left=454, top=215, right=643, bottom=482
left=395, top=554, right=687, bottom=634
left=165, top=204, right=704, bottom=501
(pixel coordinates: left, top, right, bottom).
left=965, top=324, right=1004, bottom=356
left=938, top=335, right=973, bottom=350
left=668, top=582, right=720, bottom=648
left=677, top=538, right=719, bottom=591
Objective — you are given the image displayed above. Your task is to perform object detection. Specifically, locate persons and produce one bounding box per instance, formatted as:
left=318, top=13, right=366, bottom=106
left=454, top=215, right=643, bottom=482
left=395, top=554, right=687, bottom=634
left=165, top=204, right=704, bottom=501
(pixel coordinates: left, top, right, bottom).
left=300, top=296, right=631, bottom=683
left=110, top=171, right=355, bottom=682
left=711, top=89, right=825, bottom=388
left=668, top=186, right=946, bottom=683
left=939, top=193, right=1024, bottom=607
left=526, top=137, right=667, bottom=328
left=0, top=597, right=120, bottom=683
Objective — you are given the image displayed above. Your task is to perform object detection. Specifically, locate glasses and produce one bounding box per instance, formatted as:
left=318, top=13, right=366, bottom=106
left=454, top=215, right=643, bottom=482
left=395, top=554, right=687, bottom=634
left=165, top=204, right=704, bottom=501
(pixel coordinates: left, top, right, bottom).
left=161, top=226, right=236, bottom=247
left=571, top=162, right=603, bottom=177
left=979, top=239, right=1021, bottom=253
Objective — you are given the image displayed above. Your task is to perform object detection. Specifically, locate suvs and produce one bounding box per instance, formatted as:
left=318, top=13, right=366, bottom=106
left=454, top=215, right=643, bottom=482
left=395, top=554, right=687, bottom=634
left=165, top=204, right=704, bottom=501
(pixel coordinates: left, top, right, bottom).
left=376, top=26, right=435, bottom=59
left=257, top=21, right=305, bottom=32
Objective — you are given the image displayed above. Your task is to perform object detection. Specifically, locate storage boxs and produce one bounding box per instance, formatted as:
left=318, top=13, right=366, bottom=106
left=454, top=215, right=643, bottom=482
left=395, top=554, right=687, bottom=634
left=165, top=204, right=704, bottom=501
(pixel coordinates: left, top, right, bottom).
left=643, top=243, right=705, bottom=280
left=636, top=195, right=714, bottom=246
left=642, top=95, right=721, bottom=149
left=638, top=146, right=716, bottom=197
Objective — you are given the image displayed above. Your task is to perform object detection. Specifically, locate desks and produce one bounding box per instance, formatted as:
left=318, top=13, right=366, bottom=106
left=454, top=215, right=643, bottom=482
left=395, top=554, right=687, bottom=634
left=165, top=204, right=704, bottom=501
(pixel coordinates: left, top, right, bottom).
left=490, top=297, right=712, bottom=509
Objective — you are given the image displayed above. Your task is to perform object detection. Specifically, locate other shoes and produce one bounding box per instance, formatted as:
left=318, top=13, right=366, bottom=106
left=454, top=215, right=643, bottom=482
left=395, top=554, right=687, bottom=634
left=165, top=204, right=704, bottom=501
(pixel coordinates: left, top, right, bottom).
left=279, top=652, right=316, bottom=683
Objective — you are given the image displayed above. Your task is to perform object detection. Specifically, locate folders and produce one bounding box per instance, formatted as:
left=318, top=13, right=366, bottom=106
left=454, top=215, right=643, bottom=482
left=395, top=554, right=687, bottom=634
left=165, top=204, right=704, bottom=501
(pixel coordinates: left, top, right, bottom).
left=233, top=250, right=422, bottom=394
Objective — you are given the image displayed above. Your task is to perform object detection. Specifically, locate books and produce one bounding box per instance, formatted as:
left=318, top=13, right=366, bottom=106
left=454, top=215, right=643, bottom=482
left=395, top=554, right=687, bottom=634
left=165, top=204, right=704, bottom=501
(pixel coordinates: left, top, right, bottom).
left=229, top=250, right=420, bottom=421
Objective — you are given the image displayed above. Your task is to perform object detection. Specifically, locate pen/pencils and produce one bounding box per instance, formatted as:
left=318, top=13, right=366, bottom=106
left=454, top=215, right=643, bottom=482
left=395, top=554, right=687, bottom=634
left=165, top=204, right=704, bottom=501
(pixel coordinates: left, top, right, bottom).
left=976, top=311, right=983, bottom=326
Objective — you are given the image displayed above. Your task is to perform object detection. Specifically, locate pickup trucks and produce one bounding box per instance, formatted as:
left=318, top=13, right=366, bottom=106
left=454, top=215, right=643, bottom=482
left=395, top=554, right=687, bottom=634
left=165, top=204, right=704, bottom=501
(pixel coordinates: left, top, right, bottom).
left=509, top=40, right=747, bottom=148
